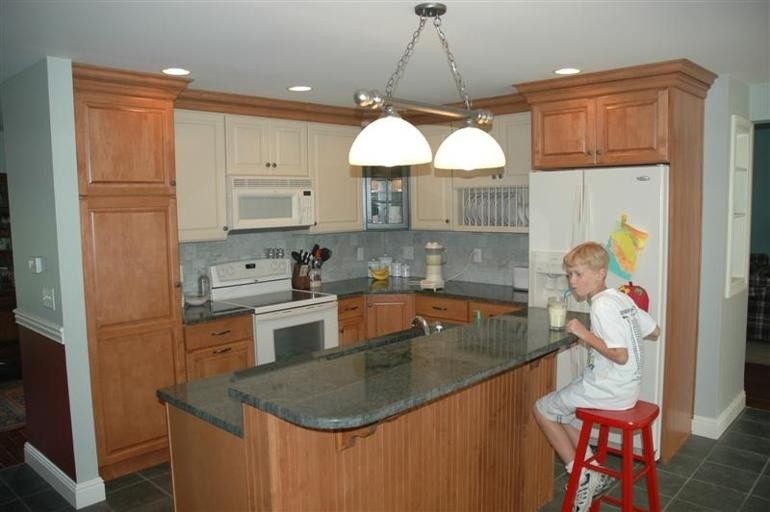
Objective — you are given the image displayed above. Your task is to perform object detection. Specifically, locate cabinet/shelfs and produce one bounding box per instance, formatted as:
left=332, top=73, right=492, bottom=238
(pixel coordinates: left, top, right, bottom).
left=360, top=159, right=411, bottom=235
left=71, top=60, right=190, bottom=483
left=172, top=105, right=229, bottom=245
left=724, top=114, right=758, bottom=304
left=362, top=290, right=417, bottom=343
left=412, top=290, right=469, bottom=329
left=512, top=56, right=719, bottom=173
left=182, top=310, right=256, bottom=385
left=448, top=110, right=533, bottom=237
left=223, top=111, right=311, bottom=180
left=337, top=291, right=366, bottom=346
left=406, top=118, right=455, bottom=234
left=468, top=298, right=522, bottom=326
left=292, top=118, right=366, bottom=238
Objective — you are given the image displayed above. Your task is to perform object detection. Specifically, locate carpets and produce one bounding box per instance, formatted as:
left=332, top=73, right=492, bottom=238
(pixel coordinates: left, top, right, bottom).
left=0, top=370, right=30, bottom=434
left=744, top=361, right=770, bottom=411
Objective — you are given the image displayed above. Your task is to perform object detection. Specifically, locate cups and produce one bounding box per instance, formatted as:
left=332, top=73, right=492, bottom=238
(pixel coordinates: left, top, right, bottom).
left=549, top=331, right=568, bottom=345
left=367, top=251, right=412, bottom=279
left=547, top=296, right=567, bottom=331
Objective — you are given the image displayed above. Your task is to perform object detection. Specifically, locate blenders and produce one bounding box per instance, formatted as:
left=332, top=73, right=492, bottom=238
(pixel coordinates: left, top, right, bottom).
left=420, top=240, right=448, bottom=292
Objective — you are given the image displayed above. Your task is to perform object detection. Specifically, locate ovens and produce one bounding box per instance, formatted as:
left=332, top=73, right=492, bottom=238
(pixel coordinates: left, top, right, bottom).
left=253, top=303, right=339, bottom=366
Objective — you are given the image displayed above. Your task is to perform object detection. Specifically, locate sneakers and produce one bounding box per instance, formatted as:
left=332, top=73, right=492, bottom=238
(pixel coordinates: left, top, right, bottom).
left=564, top=466, right=615, bottom=512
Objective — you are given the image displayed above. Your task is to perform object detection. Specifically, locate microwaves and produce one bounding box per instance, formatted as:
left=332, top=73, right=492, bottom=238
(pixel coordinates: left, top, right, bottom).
left=226, top=175, right=314, bottom=229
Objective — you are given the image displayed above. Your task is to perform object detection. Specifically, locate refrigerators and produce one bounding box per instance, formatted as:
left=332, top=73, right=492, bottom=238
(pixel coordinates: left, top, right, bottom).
left=528, top=165, right=670, bottom=466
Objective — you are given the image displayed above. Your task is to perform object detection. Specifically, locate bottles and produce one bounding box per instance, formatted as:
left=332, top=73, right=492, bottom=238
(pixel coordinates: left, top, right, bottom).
left=266, top=247, right=284, bottom=259
left=309, top=261, right=322, bottom=288
left=198, top=268, right=211, bottom=297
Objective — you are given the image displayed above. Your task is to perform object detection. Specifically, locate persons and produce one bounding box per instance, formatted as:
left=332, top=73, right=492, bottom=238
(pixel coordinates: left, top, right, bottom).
left=531, top=242, right=661, bottom=512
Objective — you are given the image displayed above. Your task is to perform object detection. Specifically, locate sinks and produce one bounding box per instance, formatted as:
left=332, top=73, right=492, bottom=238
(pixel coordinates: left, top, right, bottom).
left=315, top=324, right=446, bottom=362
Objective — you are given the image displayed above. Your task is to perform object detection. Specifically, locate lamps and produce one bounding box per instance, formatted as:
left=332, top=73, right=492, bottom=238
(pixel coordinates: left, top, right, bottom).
left=343, top=1, right=512, bottom=175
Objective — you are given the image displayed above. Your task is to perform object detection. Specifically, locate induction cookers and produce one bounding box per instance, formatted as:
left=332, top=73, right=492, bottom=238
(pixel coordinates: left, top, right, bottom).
left=208, top=279, right=339, bottom=316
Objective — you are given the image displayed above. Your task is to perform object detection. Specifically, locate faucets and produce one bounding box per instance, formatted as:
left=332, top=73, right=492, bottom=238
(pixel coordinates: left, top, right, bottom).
left=409, top=314, right=432, bottom=336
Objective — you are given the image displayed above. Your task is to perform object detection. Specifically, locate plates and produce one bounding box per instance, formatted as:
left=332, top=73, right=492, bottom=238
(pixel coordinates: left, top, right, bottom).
left=464, top=193, right=528, bottom=225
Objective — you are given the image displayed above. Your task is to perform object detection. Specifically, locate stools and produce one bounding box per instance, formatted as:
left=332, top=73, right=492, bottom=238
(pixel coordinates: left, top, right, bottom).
left=560, top=398, right=666, bottom=512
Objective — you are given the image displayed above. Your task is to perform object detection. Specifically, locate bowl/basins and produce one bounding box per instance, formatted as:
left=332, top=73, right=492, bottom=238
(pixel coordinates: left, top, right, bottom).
left=185, top=291, right=208, bottom=309
